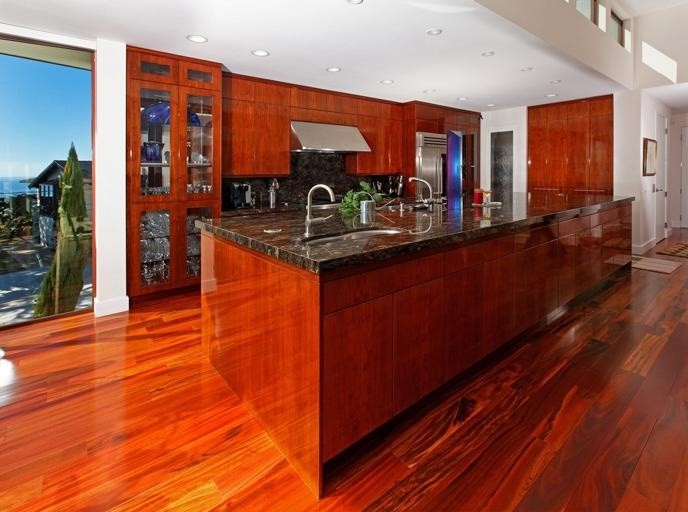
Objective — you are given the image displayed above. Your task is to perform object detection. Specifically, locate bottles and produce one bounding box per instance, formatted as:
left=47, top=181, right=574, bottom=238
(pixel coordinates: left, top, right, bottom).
left=141, top=168, right=148, bottom=195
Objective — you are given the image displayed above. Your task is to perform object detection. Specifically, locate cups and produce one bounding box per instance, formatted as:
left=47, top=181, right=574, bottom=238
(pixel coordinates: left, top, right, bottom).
left=142, top=141, right=165, bottom=163
left=473, top=189, right=483, bottom=204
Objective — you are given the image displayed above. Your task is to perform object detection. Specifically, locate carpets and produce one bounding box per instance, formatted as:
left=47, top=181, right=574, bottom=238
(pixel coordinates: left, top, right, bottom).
left=656, top=240, right=688, bottom=258
left=632, top=255, right=683, bottom=275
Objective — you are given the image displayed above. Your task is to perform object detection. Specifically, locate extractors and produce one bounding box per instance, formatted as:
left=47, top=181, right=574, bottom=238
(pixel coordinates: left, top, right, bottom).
left=290, top=120, right=372, bottom=154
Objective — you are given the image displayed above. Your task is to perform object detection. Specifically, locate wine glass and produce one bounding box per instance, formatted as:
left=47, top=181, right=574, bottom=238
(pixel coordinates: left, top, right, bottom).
left=483, top=188, right=495, bottom=206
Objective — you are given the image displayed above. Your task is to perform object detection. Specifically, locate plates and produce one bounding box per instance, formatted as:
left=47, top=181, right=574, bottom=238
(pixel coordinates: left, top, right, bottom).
left=191, top=151, right=204, bottom=161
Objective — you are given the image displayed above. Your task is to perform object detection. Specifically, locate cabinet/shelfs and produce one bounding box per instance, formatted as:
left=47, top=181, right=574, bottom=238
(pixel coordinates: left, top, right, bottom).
left=344, top=98, right=402, bottom=176
left=567, top=95, right=613, bottom=196
left=456, top=110, right=480, bottom=190
left=222, top=72, right=291, bottom=178
left=528, top=101, right=567, bottom=192
left=126, top=44, right=222, bottom=297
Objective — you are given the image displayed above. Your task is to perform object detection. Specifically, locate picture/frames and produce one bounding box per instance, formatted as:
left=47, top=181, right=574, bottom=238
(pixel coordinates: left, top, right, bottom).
left=643, top=138, right=657, bottom=176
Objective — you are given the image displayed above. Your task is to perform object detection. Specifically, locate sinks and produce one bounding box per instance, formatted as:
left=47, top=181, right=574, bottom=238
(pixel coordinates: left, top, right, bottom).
left=303, top=227, right=402, bottom=248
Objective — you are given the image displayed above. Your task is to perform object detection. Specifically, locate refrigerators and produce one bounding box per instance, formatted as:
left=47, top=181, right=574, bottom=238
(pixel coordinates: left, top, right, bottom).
left=415, top=131, right=448, bottom=196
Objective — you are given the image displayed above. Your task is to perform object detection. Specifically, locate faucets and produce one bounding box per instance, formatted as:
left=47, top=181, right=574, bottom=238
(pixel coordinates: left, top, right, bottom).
left=408, top=215, right=433, bottom=234
left=305, top=183, right=335, bottom=234
left=409, top=176, right=433, bottom=204
